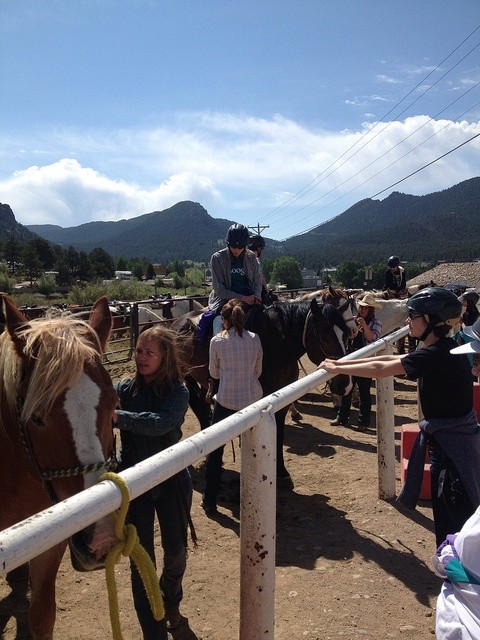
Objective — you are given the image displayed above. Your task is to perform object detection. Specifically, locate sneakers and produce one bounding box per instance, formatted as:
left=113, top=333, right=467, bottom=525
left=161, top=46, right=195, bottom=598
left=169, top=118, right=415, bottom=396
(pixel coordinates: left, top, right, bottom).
left=162, top=600, right=182, bottom=629
left=200, top=501, right=218, bottom=510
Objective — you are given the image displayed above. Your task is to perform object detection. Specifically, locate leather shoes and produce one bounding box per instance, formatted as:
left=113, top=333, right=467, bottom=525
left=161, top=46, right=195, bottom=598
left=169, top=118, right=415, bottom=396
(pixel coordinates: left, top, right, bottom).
left=357, top=423, right=367, bottom=431
left=330, top=418, right=350, bottom=426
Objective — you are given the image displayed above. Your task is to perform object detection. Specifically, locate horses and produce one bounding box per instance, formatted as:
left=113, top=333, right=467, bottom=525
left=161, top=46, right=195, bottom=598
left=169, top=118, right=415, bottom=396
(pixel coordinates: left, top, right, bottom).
left=332, top=298, right=409, bottom=408
left=138, top=296, right=204, bottom=321
left=169, top=298, right=353, bottom=493
left=350, top=280, right=436, bottom=354
left=109, top=306, right=169, bottom=337
left=278, top=285, right=359, bottom=420
left=0, top=295, right=120, bottom=640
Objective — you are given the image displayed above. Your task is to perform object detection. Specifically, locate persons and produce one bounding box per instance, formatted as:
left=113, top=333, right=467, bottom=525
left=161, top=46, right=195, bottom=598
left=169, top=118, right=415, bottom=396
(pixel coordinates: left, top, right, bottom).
left=246, top=234, right=265, bottom=257
left=205, top=224, right=263, bottom=405
left=331, top=295, right=383, bottom=432
left=458, top=291, right=480, bottom=383
left=317, top=286, right=480, bottom=552
left=108, top=326, right=193, bottom=639
left=385, top=255, right=407, bottom=299
left=199, top=298, right=264, bottom=518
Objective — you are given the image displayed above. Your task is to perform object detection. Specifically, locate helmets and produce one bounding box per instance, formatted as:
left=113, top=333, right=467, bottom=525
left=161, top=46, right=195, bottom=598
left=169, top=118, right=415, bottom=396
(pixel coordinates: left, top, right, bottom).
left=225, top=223, right=249, bottom=245
left=249, top=236, right=265, bottom=246
left=463, top=292, right=480, bottom=301
left=387, top=255, right=400, bottom=266
left=406, top=288, right=461, bottom=319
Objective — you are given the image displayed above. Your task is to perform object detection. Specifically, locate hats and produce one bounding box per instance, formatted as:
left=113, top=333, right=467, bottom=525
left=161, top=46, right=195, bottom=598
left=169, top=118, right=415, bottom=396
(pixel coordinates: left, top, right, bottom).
left=357, top=293, right=382, bottom=307
